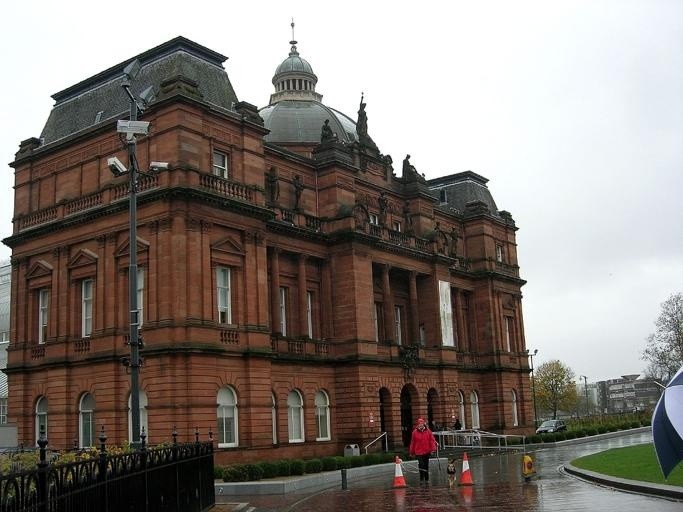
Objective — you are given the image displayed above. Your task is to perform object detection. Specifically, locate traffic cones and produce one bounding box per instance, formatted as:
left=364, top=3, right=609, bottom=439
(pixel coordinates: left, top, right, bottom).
left=391, top=455, right=409, bottom=489
left=457, top=451, right=475, bottom=486
left=520, top=455, right=538, bottom=478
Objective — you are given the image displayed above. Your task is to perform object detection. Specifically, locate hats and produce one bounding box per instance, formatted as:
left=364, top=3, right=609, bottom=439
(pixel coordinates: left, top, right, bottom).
left=417, top=418, right=425, bottom=424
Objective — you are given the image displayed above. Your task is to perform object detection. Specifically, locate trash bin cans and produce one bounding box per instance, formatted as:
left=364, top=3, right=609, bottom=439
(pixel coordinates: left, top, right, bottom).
left=344, top=444, right=360, bottom=456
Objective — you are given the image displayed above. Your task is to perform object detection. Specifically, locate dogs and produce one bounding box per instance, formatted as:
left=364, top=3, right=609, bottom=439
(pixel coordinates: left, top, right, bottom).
left=447, top=454, right=458, bottom=489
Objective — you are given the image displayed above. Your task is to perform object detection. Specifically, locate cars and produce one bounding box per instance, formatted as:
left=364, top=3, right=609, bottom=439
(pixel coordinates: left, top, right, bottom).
left=535, top=420, right=567, bottom=436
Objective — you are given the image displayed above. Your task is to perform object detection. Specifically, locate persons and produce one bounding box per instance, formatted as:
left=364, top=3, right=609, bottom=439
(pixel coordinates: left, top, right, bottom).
left=455, top=419, right=462, bottom=429
left=379, top=192, right=389, bottom=223
left=410, top=417, right=437, bottom=485
left=266, top=164, right=280, bottom=203
left=452, top=226, right=459, bottom=255
left=436, top=221, right=440, bottom=239
left=356, top=92, right=368, bottom=136
left=293, top=173, right=304, bottom=208
left=320, top=119, right=332, bottom=143
left=403, top=154, right=410, bottom=178
left=404, top=199, right=413, bottom=234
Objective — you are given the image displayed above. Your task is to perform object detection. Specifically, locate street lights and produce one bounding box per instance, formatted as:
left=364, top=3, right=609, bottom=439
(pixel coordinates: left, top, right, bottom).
left=526, top=349, right=538, bottom=425
left=107, top=58, right=173, bottom=459
left=579, top=375, right=589, bottom=418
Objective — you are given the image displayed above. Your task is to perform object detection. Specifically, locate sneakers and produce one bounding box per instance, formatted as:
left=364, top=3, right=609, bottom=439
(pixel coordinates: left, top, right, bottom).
left=419, top=479, right=430, bottom=485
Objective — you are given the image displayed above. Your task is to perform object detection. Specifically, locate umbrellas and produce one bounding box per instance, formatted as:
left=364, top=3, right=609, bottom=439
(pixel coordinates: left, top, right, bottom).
left=650, top=367, right=683, bottom=480
